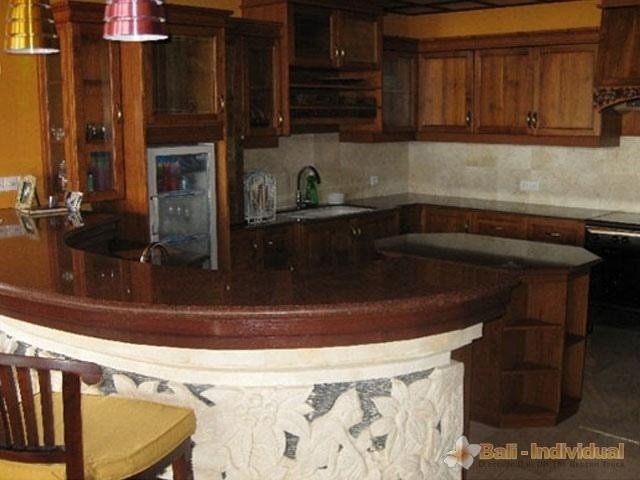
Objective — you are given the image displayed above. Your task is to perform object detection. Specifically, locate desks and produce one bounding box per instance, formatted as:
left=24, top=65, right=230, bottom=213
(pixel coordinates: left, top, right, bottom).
left=373, top=232, right=604, bottom=430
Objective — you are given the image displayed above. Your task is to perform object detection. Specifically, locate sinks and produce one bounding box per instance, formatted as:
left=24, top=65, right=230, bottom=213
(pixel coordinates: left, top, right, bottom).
left=285, top=205, right=373, bottom=219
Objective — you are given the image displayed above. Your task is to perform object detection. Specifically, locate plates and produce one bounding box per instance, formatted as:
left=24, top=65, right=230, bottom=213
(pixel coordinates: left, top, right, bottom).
left=326, top=191, right=345, bottom=206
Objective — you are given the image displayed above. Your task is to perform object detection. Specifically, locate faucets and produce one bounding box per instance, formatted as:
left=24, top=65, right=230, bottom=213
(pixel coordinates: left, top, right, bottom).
left=140, top=242, right=172, bottom=269
left=296, top=165, right=321, bottom=208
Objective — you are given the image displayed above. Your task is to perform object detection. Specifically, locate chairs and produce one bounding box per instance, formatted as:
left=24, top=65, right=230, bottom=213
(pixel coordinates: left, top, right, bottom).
left=0, top=353, right=196, bottom=480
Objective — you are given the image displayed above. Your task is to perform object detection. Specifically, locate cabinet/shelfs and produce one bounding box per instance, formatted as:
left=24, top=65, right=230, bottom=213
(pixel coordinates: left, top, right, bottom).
left=138, top=3, right=233, bottom=125
left=290, top=6, right=382, bottom=133
left=372, top=204, right=420, bottom=243
left=234, top=222, right=303, bottom=272
left=422, top=207, right=529, bottom=238
left=474, top=44, right=622, bottom=135
left=382, top=37, right=415, bottom=130
left=304, top=210, right=371, bottom=269
left=150, top=141, right=219, bottom=274
left=42, top=5, right=121, bottom=199
left=529, top=216, right=584, bottom=246
left=414, top=47, right=474, bottom=130
left=238, top=21, right=291, bottom=139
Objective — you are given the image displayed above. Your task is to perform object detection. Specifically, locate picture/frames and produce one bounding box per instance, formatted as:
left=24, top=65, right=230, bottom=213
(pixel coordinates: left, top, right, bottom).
left=14, top=175, right=37, bottom=212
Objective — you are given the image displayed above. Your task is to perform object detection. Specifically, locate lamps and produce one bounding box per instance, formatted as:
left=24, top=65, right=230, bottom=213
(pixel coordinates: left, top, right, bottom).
left=1, top=1, right=64, bottom=57
left=101, top=1, right=169, bottom=45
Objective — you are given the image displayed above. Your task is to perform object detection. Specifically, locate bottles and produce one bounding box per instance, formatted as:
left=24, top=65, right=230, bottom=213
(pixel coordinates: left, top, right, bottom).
left=157, top=156, right=208, bottom=239
left=305, top=174, right=319, bottom=208
left=49, top=123, right=115, bottom=196
